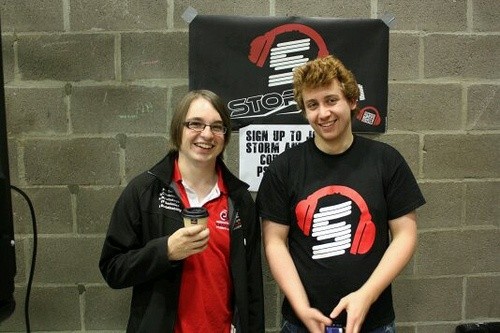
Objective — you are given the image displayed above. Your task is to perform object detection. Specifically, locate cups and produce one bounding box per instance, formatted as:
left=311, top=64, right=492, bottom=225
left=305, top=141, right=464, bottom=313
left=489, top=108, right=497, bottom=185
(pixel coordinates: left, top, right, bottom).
left=180, top=207, right=210, bottom=230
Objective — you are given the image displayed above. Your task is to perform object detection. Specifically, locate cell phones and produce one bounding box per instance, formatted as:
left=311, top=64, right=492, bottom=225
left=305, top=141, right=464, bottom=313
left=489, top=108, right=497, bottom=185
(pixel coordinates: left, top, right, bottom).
left=325, top=316, right=343, bottom=333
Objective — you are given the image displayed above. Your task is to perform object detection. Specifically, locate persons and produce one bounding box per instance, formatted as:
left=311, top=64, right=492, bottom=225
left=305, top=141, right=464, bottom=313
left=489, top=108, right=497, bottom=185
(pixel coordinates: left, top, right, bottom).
left=98, top=88, right=265, bottom=333
left=256, top=57, right=425, bottom=333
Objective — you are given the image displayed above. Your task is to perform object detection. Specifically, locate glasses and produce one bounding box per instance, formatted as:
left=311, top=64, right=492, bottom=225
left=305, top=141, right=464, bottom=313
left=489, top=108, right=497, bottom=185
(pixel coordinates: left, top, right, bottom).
left=183, top=121, right=227, bottom=135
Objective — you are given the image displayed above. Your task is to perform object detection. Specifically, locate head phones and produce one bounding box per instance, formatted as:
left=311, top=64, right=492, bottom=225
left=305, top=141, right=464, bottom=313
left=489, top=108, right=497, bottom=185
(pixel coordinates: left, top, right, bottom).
left=248, top=22, right=330, bottom=69
left=356, top=106, right=382, bottom=126
left=295, top=184, right=375, bottom=255
